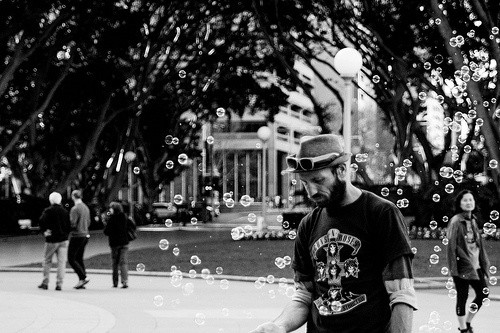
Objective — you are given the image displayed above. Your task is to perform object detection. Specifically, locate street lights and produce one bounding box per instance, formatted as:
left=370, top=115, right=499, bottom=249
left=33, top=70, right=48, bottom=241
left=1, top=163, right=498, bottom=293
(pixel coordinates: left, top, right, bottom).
left=257, top=126, right=273, bottom=226
left=333, top=47, right=363, bottom=183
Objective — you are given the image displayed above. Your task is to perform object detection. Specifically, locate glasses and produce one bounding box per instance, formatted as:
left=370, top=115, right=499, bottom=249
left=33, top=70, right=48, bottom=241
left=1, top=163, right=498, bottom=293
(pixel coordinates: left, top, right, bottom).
left=285, top=153, right=340, bottom=171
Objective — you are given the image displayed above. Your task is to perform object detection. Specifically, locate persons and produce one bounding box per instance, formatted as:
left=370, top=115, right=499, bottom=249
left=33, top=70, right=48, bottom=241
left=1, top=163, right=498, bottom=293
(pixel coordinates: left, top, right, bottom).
left=68, top=190, right=91, bottom=288
left=37, top=191, right=72, bottom=290
left=251, top=135, right=419, bottom=333
left=445, top=189, right=491, bottom=333
left=103, top=202, right=137, bottom=288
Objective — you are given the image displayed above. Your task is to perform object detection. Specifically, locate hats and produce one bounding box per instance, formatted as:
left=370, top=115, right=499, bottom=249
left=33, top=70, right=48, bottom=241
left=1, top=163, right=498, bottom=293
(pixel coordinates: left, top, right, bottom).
left=284, top=133, right=350, bottom=173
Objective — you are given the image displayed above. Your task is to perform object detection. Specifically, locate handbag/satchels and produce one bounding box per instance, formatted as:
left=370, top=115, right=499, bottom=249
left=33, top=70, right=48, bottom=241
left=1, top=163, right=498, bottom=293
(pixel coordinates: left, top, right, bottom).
left=128, top=230, right=138, bottom=240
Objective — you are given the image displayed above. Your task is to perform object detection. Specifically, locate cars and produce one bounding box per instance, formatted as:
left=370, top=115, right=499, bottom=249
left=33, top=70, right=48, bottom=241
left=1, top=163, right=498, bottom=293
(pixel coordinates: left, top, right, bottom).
left=154, top=203, right=216, bottom=224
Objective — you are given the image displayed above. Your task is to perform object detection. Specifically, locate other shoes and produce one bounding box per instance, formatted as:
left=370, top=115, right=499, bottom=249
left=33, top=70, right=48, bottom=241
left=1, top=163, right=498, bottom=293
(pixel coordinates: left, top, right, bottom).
left=56, top=286, right=61, bottom=290
left=38, top=284, right=48, bottom=289
left=121, top=283, right=128, bottom=288
left=458, top=322, right=474, bottom=333
left=113, top=284, right=118, bottom=287
left=73, top=279, right=89, bottom=289
